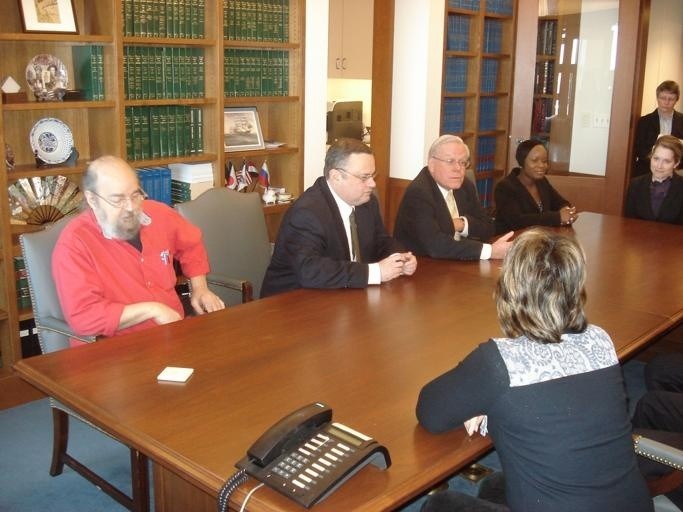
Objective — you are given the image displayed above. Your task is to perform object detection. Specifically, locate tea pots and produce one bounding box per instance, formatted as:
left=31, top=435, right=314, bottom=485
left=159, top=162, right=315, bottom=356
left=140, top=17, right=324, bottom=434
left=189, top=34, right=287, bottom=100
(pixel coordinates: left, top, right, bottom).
left=262, top=187, right=276, bottom=206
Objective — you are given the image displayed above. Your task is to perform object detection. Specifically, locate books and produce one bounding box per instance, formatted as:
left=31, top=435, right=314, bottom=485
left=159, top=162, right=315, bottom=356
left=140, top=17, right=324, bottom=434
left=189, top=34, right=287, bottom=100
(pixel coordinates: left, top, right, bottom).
left=121, top=0, right=292, bottom=158
left=442, top=0, right=561, bottom=217
left=12, top=254, right=33, bottom=310
left=70, top=43, right=98, bottom=100
left=97, top=43, right=106, bottom=101
left=135, top=164, right=214, bottom=207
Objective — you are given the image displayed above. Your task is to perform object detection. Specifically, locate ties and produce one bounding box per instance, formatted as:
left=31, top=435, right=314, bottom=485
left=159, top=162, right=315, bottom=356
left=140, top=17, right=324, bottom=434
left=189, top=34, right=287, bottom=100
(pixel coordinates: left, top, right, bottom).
left=446, top=190, right=462, bottom=241
left=350, top=207, right=361, bottom=263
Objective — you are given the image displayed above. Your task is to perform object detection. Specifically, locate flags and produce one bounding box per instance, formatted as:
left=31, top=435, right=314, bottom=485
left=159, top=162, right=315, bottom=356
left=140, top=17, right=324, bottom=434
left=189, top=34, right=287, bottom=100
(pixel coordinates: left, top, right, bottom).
left=224, top=158, right=271, bottom=194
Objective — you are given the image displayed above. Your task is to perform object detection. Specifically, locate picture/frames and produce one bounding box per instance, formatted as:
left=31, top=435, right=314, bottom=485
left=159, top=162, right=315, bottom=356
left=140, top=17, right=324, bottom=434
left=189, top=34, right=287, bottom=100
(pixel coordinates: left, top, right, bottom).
left=16, top=0, right=80, bottom=35
left=223, top=106, right=266, bottom=153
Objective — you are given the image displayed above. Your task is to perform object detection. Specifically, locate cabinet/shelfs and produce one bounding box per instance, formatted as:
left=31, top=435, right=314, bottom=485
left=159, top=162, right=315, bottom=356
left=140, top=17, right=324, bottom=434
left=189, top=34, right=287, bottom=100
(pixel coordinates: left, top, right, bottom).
left=326, top=0, right=374, bottom=81
left=0, top=0, right=306, bottom=408
left=438, top=0, right=518, bottom=212
left=530, top=12, right=582, bottom=176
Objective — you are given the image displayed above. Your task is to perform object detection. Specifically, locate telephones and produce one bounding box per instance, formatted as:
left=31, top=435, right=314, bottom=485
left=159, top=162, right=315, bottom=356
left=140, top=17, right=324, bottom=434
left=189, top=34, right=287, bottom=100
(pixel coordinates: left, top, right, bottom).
left=235, top=402, right=392, bottom=509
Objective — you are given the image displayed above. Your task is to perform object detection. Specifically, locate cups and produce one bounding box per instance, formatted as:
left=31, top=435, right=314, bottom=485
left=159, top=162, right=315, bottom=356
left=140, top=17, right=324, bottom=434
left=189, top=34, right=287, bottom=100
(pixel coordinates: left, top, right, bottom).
left=278, top=193, right=291, bottom=202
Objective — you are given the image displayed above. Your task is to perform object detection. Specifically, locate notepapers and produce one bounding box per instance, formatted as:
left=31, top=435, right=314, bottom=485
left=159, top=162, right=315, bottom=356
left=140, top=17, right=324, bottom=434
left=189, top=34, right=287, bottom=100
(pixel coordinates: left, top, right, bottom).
left=157, top=365, right=194, bottom=383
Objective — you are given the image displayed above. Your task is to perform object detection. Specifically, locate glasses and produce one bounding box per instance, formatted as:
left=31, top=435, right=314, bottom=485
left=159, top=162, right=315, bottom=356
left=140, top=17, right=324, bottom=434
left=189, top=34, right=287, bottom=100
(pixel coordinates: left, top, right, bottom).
left=432, top=157, right=470, bottom=169
left=335, top=167, right=379, bottom=182
left=88, top=187, right=149, bottom=208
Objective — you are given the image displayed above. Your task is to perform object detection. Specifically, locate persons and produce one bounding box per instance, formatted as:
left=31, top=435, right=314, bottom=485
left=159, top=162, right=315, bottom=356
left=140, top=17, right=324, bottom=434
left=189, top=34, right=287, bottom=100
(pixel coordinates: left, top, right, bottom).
left=392, top=133, right=517, bottom=262
left=50, top=151, right=226, bottom=351
left=257, top=135, right=418, bottom=300
left=631, top=349, right=682, bottom=483
left=619, top=134, right=682, bottom=228
left=412, top=225, right=657, bottom=512
left=633, top=80, right=682, bottom=176
left=492, top=138, right=579, bottom=240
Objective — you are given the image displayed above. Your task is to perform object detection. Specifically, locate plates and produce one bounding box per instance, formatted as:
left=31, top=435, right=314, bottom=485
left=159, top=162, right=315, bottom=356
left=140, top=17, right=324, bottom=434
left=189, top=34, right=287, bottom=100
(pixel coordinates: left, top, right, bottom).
left=30, top=118, right=74, bottom=165
left=25, top=54, right=70, bottom=100
